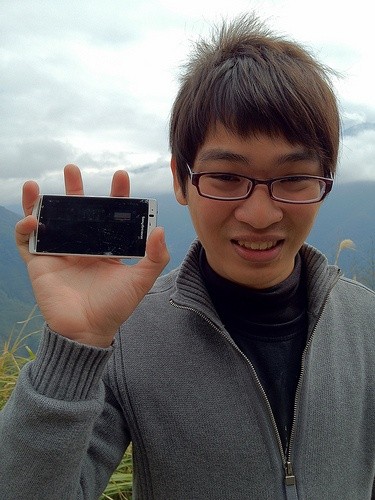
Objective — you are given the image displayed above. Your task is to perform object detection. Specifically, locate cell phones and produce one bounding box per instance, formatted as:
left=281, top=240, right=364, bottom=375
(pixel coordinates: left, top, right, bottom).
left=29, top=194, right=158, bottom=259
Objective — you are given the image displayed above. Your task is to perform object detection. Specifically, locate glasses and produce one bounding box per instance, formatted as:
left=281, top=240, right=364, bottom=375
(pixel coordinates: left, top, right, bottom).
left=184, top=161, right=334, bottom=205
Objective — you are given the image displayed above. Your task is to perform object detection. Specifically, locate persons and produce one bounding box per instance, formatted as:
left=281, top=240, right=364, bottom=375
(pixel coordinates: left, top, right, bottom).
left=1, top=13, right=375, bottom=500
